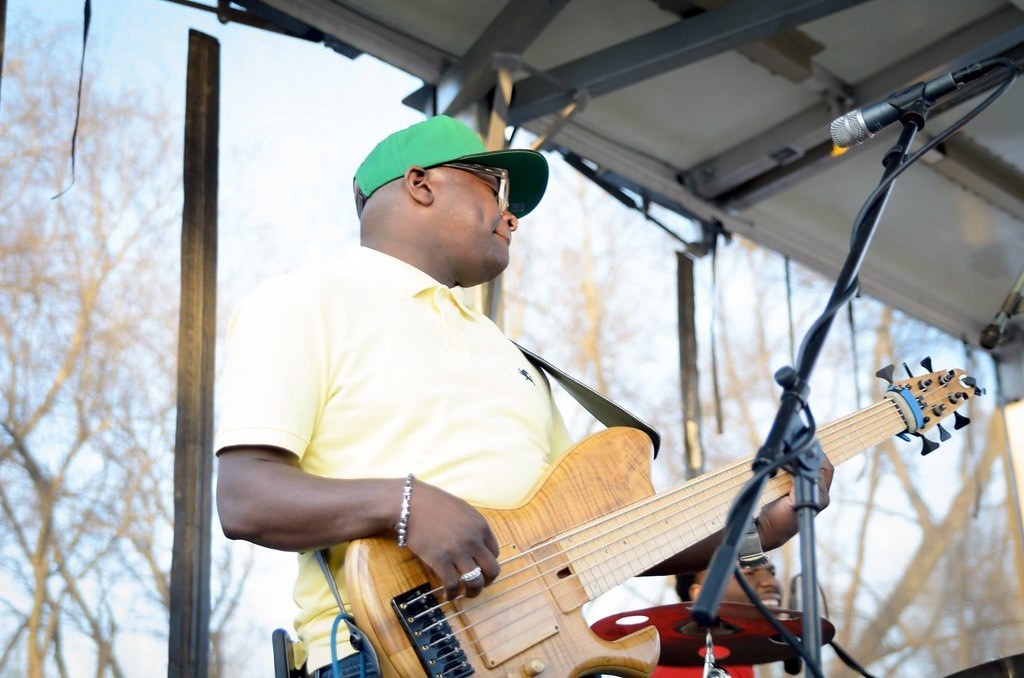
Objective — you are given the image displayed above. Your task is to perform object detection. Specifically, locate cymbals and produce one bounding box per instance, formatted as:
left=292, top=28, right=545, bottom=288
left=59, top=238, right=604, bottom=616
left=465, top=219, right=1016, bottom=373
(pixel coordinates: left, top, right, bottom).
left=941, top=651, right=1024, bottom=678
left=590, top=601, right=836, bottom=667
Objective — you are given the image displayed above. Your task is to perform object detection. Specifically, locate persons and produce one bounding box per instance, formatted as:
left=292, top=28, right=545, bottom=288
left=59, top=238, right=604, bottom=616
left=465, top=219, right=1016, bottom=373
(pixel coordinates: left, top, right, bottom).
left=214, top=116, right=832, bottom=678
left=673, top=544, right=782, bottom=616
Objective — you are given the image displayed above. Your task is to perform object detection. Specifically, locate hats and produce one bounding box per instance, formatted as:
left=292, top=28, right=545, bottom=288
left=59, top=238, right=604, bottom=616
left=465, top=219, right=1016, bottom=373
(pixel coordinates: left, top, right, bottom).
left=349, top=113, right=550, bottom=218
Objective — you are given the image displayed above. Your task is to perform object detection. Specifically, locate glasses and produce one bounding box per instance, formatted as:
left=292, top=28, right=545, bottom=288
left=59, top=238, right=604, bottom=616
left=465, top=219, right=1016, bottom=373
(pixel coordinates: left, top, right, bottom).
left=438, top=162, right=512, bottom=212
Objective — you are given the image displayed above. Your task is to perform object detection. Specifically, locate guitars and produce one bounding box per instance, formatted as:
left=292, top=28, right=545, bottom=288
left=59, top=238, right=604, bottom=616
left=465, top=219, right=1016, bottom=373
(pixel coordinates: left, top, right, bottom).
left=344, top=354, right=986, bottom=678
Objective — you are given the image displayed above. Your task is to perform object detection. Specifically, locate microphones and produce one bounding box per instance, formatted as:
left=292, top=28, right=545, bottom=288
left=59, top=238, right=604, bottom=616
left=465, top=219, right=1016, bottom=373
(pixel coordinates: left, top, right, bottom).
left=830, top=55, right=1000, bottom=149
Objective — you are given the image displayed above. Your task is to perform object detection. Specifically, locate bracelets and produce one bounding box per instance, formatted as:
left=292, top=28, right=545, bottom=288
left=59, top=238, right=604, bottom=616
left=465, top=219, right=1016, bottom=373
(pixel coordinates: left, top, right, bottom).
left=399, top=475, right=414, bottom=544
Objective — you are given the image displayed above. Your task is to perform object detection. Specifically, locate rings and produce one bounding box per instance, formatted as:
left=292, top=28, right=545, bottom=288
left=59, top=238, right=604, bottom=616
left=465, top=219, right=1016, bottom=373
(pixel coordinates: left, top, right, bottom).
left=458, top=566, right=482, bottom=581
